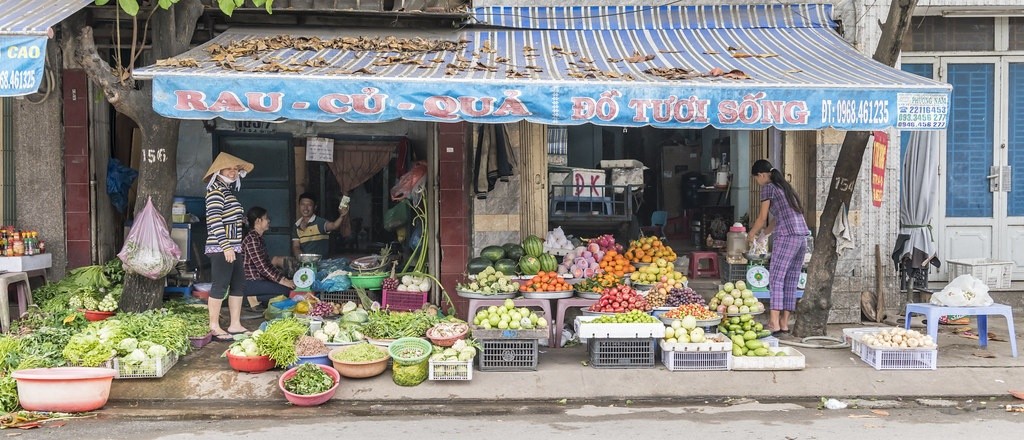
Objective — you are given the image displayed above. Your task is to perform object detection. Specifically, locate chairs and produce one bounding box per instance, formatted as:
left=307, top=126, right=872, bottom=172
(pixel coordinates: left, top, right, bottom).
left=639, top=210, right=670, bottom=241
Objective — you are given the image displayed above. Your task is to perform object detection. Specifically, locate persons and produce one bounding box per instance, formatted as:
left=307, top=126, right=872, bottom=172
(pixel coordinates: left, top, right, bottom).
left=237, top=205, right=297, bottom=312
left=743, top=159, right=813, bottom=336
left=292, top=190, right=350, bottom=264
left=200, top=152, right=255, bottom=344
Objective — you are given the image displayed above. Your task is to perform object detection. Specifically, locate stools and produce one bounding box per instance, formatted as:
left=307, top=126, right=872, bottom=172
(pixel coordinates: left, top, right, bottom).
left=1, top=272, right=36, bottom=332
left=689, top=250, right=720, bottom=280
left=905, top=299, right=1018, bottom=358
left=467, top=297, right=601, bottom=349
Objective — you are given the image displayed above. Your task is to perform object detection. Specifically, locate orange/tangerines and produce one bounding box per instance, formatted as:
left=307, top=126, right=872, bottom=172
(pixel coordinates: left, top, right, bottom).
left=600, top=249, right=636, bottom=277
left=624, top=236, right=677, bottom=263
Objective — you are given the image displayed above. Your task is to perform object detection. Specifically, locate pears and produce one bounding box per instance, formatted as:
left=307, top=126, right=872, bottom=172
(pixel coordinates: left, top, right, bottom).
left=473, top=299, right=547, bottom=330
left=631, top=258, right=687, bottom=285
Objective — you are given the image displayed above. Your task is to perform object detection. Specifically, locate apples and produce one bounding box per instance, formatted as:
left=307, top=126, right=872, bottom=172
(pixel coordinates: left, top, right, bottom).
left=588, top=284, right=652, bottom=313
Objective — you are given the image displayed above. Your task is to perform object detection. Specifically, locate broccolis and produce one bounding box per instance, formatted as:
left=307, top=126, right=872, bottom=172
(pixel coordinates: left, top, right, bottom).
left=456, top=265, right=520, bottom=293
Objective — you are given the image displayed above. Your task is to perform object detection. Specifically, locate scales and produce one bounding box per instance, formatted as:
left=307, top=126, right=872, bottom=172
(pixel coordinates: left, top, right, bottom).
left=797, top=264, right=808, bottom=289
left=292, top=254, right=323, bottom=292
left=742, top=251, right=772, bottom=291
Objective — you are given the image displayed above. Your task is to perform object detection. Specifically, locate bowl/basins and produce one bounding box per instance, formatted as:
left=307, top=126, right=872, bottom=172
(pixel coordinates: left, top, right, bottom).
left=349, top=255, right=390, bottom=290
left=11, top=366, right=119, bottom=412
left=226, top=317, right=469, bottom=407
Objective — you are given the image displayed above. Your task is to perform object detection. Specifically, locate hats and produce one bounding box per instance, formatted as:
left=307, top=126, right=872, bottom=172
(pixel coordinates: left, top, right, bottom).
left=202, top=151, right=255, bottom=183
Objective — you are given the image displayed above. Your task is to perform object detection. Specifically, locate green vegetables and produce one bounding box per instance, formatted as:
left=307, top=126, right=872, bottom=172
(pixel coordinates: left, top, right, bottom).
left=0, top=255, right=211, bottom=413
left=361, top=311, right=432, bottom=339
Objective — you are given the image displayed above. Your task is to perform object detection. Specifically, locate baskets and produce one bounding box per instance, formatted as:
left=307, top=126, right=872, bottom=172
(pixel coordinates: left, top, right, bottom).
left=387, top=336, right=432, bottom=366
left=426, top=322, right=469, bottom=347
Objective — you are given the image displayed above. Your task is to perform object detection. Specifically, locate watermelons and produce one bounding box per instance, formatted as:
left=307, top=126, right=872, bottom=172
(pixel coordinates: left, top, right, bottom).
left=467, top=235, right=558, bottom=276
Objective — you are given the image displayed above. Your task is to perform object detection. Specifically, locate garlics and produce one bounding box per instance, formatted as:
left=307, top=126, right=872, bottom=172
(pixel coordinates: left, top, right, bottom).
left=862, top=323, right=933, bottom=348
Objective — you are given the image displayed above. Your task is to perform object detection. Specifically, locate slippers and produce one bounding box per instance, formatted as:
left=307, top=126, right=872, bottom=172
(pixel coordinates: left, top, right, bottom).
left=246, top=302, right=266, bottom=313
left=227, top=330, right=252, bottom=336
left=762, top=323, right=791, bottom=334
left=210, top=334, right=234, bottom=341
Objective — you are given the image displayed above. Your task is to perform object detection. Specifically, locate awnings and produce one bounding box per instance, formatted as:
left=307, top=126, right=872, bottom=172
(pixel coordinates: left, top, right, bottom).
left=119, top=5, right=959, bottom=133
left=0, top=0, right=96, bottom=99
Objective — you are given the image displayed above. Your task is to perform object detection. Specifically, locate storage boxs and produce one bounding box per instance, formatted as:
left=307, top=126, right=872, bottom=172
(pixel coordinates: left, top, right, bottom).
left=477, top=336, right=540, bottom=372
left=718, top=254, right=748, bottom=285
left=662, top=344, right=730, bottom=371
left=382, top=286, right=428, bottom=310
left=586, top=337, right=657, bottom=369
left=945, top=257, right=1016, bottom=290
left=319, top=289, right=381, bottom=308
left=109, top=346, right=177, bottom=379
left=428, top=353, right=474, bottom=382
left=851, top=330, right=936, bottom=372
left=188, top=331, right=213, bottom=348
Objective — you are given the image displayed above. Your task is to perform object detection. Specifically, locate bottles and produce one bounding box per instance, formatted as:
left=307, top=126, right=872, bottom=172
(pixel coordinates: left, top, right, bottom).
left=716, top=165, right=728, bottom=189
left=0, top=226, right=41, bottom=256
left=727, top=221, right=750, bottom=259
